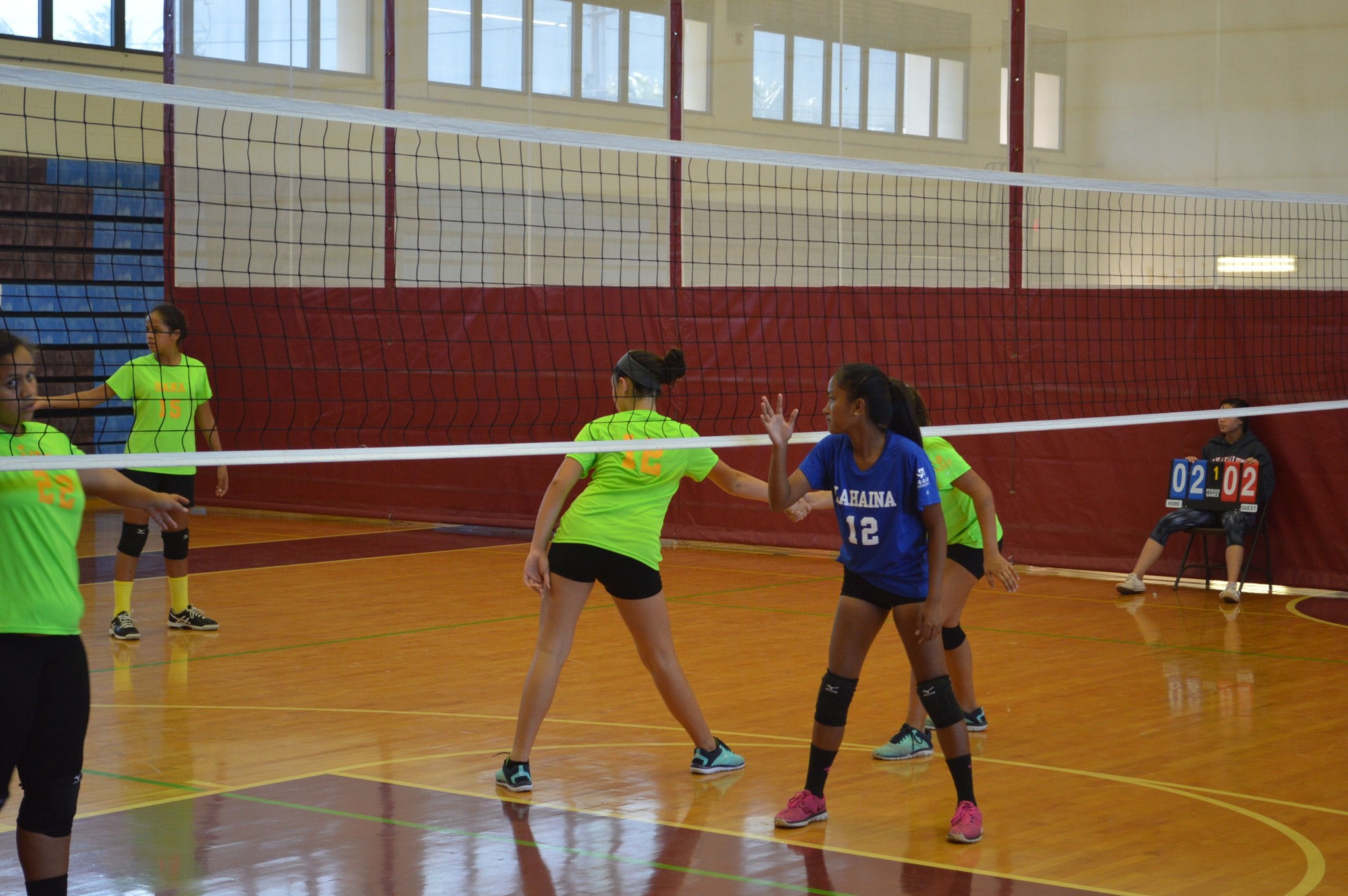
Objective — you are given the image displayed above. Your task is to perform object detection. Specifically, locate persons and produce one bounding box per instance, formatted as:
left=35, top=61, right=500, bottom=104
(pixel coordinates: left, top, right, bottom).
left=758, top=362, right=986, bottom=844
left=1114, top=396, right=1276, bottom=604
left=31, top=304, right=231, bottom=642
left=493, top=346, right=812, bottom=793
left=783, top=376, right=1022, bottom=763
left=0, top=324, right=192, bottom=895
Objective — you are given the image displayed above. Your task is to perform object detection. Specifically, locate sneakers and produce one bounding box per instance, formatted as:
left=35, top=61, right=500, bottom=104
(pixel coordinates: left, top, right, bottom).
left=492, top=752, right=533, bottom=792
left=774, top=789, right=828, bottom=827
left=925, top=706, right=988, bottom=732
left=872, top=723, right=934, bottom=760
left=1115, top=573, right=1146, bottom=594
left=1219, top=581, right=1241, bottom=603
left=167, top=602, right=220, bottom=631
left=690, top=736, right=745, bottom=774
left=946, top=801, right=983, bottom=843
left=108, top=609, right=141, bottom=641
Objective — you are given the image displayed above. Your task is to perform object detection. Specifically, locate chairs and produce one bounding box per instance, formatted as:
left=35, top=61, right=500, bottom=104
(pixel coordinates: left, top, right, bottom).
left=1173, top=487, right=1275, bottom=597
